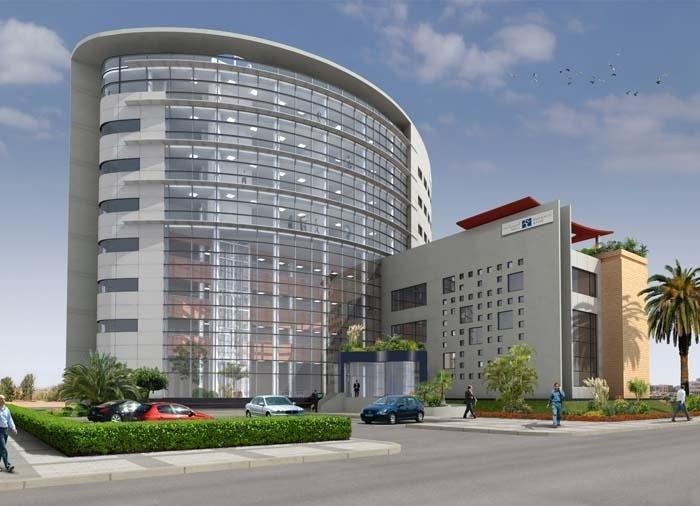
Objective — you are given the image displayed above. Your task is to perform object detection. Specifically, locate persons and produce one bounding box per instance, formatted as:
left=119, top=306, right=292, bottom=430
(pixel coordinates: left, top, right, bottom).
left=0, top=394, right=20, bottom=474
left=311, top=388, right=319, bottom=412
left=462, top=384, right=478, bottom=419
left=353, top=379, right=360, bottom=397
left=670, top=384, right=693, bottom=422
left=545, top=382, right=566, bottom=428
